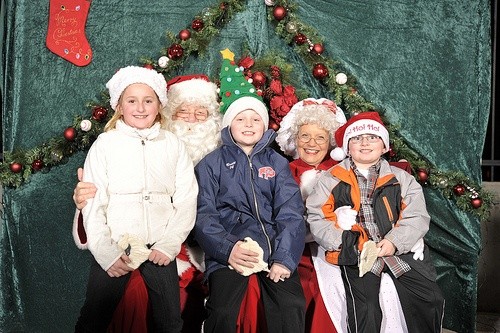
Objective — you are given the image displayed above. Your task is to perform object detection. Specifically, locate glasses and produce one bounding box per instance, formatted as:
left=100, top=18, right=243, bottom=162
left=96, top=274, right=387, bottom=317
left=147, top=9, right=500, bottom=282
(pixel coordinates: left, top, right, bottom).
left=174, top=112, right=208, bottom=120
left=350, top=135, right=381, bottom=144
left=297, top=132, right=329, bottom=144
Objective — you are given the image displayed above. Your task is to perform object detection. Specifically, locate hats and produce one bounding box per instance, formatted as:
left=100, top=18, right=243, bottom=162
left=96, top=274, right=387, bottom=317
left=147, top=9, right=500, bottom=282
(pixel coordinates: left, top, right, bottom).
left=167, top=74, right=217, bottom=97
left=330, top=112, right=390, bottom=162
left=106, top=66, right=167, bottom=110
left=217, top=48, right=269, bottom=131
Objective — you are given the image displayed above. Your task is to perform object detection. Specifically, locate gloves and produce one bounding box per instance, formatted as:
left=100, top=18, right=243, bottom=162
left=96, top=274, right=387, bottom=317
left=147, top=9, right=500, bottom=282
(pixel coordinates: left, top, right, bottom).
left=335, top=206, right=357, bottom=231
left=410, top=237, right=424, bottom=260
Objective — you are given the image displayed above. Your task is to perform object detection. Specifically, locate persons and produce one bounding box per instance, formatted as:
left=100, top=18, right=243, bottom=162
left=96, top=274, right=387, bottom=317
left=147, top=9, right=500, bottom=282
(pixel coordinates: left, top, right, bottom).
left=71, top=65, right=444, bottom=333
